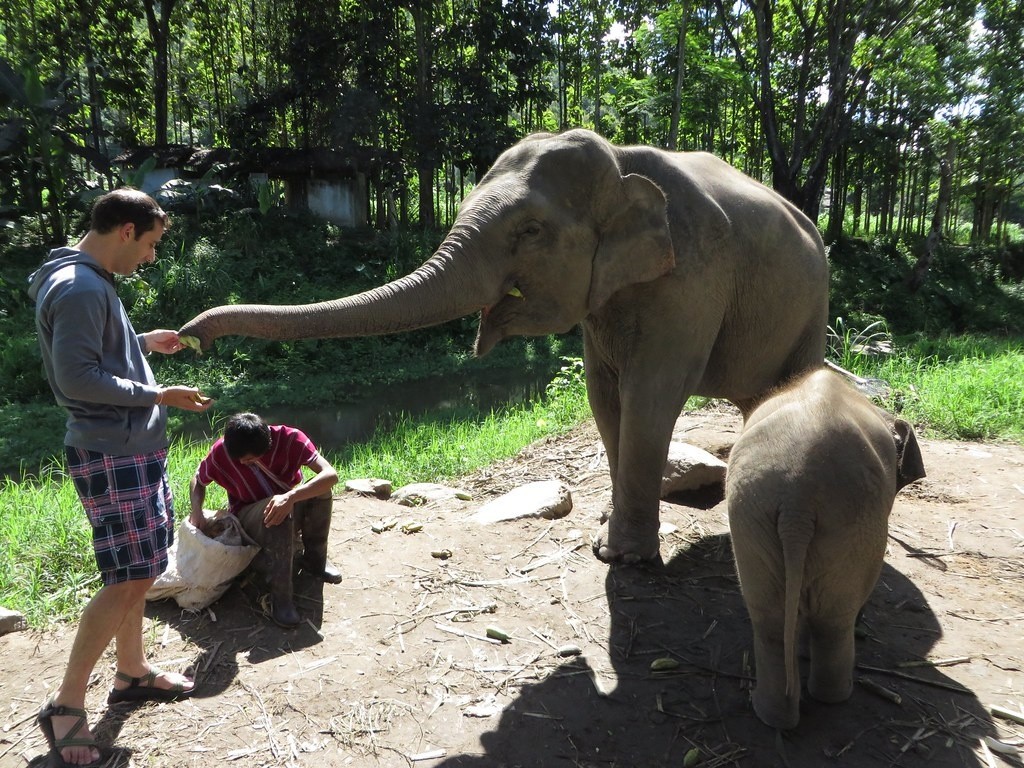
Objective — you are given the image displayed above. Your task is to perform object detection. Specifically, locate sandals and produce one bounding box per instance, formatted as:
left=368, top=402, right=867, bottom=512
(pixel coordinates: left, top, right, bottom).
left=111, top=665, right=198, bottom=699
left=38, top=694, right=103, bottom=768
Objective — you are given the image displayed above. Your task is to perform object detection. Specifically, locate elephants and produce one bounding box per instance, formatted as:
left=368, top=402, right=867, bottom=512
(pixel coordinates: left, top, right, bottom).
left=725, top=370, right=932, bottom=723
left=176, top=127, right=829, bottom=562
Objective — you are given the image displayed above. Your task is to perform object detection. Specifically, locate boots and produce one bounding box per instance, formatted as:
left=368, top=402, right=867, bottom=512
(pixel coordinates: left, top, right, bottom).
left=262, top=517, right=302, bottom=628
left=298, top=496, right=342, bottom=584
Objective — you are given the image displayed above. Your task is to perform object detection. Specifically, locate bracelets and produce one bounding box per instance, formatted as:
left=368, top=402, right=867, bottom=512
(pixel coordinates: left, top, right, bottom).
left=157, top=390, right=163, bottom=406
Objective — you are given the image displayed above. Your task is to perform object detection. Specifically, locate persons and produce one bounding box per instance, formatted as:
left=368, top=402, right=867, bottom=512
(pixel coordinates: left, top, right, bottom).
left=27, top=189, right=214, bottom=768
left=189, top=412, right=343, bottom=627
